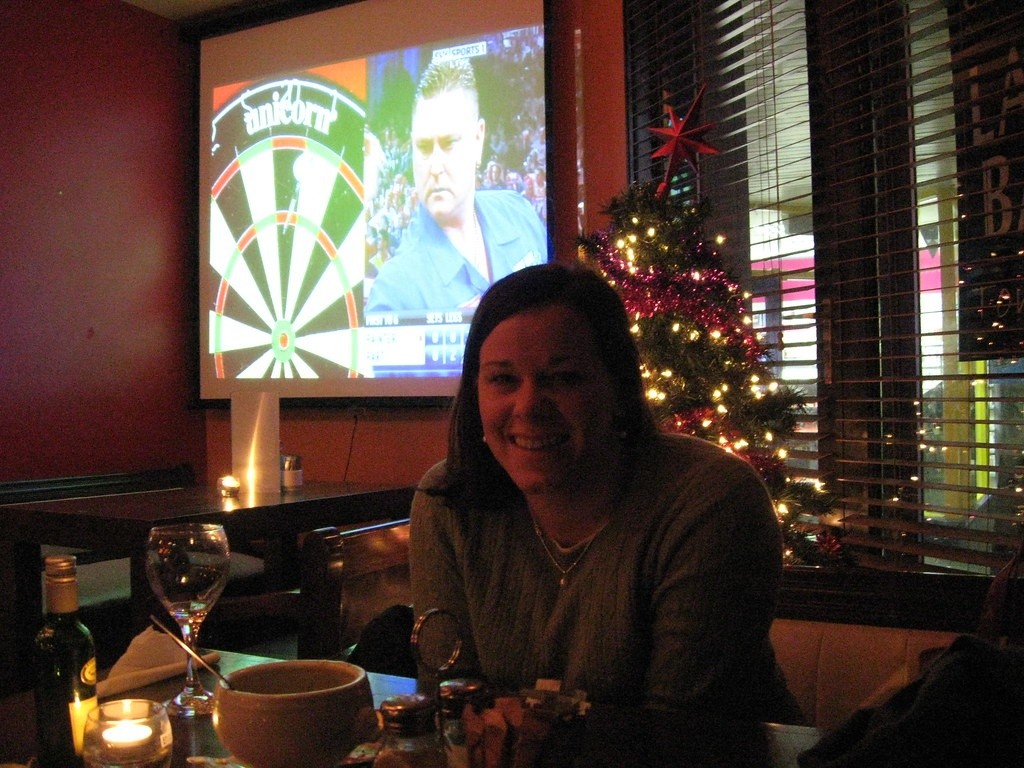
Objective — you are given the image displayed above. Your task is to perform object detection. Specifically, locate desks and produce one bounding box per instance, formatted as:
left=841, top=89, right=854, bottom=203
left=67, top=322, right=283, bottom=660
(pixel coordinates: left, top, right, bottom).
left=0, top=481, right=420, bottom=695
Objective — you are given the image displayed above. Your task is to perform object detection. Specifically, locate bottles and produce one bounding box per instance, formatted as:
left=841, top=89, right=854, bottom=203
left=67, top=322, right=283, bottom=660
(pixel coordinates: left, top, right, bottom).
left=373, top=696, right=441, bottom=768
left=30, top=555, right=97, bottom=768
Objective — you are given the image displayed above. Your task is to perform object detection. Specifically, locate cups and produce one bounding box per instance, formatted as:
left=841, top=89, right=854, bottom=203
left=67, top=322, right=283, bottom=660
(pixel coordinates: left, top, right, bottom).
left=84, top=699, right=172, bottom=768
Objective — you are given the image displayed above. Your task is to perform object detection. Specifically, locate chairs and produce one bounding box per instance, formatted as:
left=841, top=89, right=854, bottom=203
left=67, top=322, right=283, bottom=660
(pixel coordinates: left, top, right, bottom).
left=299, top=520, right=1023, bottom=728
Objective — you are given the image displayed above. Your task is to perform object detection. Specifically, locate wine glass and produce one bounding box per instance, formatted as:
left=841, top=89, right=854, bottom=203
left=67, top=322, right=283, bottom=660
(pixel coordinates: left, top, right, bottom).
left=149, top=523, right=230, bottom=717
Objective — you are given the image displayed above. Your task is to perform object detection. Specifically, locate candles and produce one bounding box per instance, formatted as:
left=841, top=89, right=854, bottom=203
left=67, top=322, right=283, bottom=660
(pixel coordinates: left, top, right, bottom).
left=82, top=700, right=174, bottom=768
left=217, top=477, right=239, bottom=497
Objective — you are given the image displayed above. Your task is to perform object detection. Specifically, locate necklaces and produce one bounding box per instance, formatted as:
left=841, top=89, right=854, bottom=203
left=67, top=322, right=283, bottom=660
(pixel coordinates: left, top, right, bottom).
left=525, top=508, right=609, bottom=589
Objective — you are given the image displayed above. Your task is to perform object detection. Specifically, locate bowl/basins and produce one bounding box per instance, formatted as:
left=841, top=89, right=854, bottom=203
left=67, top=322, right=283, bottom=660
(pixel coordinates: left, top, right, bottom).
left=213, top=660, right=368, bottom=768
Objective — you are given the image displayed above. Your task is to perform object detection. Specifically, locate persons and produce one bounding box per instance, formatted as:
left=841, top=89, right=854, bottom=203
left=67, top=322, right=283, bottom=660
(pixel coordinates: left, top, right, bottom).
left=361, top=51, right=547, bottom=320
left=406, top=260, right=807, bottom=725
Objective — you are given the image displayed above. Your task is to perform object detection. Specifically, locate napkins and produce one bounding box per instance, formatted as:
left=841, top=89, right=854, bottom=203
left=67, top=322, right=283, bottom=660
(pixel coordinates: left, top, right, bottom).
left=92, top=624, right=221, bottom=698
